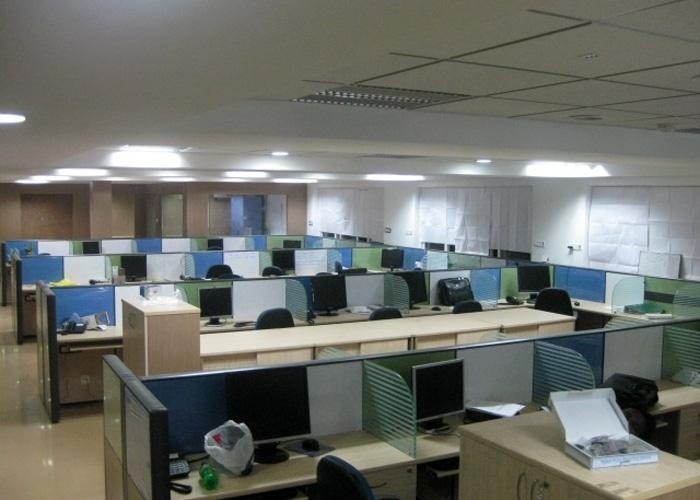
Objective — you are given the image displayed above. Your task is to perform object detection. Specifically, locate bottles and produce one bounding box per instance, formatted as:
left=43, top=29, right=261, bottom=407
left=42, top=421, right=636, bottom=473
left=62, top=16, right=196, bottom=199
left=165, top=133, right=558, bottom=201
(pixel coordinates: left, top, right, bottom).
left=200, top=461, right=219, bottom=490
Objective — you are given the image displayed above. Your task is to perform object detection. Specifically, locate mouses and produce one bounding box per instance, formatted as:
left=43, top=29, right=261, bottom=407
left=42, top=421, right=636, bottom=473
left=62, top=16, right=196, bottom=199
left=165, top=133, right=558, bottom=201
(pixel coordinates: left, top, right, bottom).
left=430, top=306, right=442, bottom=311
left=303, top=439, right=319, bottom=451
left=574, top=300, right=582, bottom=307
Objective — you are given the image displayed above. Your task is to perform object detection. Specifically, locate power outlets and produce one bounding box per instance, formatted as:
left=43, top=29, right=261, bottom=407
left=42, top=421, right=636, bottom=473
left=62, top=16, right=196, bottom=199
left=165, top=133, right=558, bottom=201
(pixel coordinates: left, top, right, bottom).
left=80, top=375, right=90, bottom=386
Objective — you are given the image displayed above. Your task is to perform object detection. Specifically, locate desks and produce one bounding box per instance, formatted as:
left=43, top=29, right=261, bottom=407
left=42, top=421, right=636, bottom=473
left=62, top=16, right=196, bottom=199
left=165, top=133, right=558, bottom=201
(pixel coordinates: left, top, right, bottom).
left=42, top=281, right=176, bottom=424
left=289, top=322, right=411, bottom=362
left=467, top=307, right=578, bottom=343
left=613, top=276, right=700, bottom=330
left=197, top=329, right=314, bottom=373
left=534, top=323, right=700, bottom=462
left=363, top=338, right=596, bottom=498
left=123, top=356, right=416, bottom=500
left=15, top=254, right=113, bottom=346
left=70, top=239, right=132, bottom=255
left=370, top=313, right=503, bottom=352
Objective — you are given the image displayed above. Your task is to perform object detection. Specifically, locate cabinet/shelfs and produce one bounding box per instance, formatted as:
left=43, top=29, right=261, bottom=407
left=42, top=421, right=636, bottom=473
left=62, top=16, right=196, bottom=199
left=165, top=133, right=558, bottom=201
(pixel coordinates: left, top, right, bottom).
left=123, top=298, right=199, bottom=378
left=458, top=409, right=699, bottom=500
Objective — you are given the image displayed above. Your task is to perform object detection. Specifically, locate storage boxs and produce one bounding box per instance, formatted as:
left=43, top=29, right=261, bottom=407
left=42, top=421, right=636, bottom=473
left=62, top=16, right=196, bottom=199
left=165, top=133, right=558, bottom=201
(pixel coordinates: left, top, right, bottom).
left=548, top=387, right=660, bottom=471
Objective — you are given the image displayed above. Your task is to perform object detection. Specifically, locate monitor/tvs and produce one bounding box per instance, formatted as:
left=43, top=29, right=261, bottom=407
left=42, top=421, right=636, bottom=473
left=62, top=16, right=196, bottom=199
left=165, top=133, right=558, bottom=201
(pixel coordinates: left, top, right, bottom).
left=82, top=242, right=99, bottom=254
left=208, top=239, right=223, bottom=250
left=121, top=256, right=146, bottom=282
left=518, top=266, right=551, bottom=304
left=200, top=288, right=232, bottom=326
left=381, top=249, right=404, bottom=271
left=391, top=272, right=427, bottom=310
left=283, top=240, right=301, bottom=248
left=272, top=250, right=295, bottom=275
left=311, top=276, right=347, bottom=316
left=225, top=366, right=311, bottom=464
left=412, top=358, right=465, bottom=434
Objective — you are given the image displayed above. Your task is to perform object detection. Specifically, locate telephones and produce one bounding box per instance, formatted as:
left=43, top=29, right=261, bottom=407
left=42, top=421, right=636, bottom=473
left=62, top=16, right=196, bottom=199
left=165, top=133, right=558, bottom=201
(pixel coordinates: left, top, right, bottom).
left=505, top=295, right=525, bottom=306
left=308, top=308, right=316, bottom=325
left=169, top=450, right=193, bottom=497
left=61, top=321, right=86, bottom=335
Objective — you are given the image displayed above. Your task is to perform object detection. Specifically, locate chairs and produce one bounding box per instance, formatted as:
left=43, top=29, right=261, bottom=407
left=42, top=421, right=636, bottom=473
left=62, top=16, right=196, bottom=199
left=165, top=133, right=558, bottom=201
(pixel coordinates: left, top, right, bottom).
left=260, top=266, right=284, bottom=277
left=535, top=287, right=574, bottom=317
left=335, top=261, right=369, bottom=276
left=316, top=455, right=378, bottom=500
left=255, top=308, right=295, bottom=329
left=451, top=302, right=484, bottom=314
left=203, top=264, right=243, bottom=282
